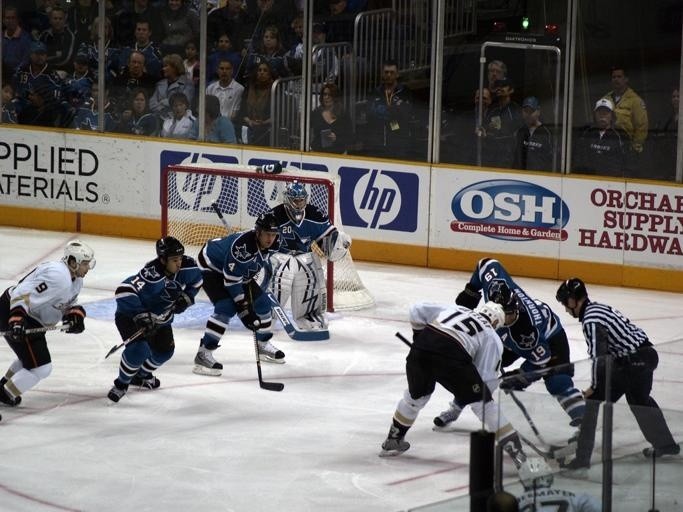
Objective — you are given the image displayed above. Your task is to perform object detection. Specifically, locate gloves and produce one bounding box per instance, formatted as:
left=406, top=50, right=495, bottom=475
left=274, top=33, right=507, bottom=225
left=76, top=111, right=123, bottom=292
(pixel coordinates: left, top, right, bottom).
left=173, top=291, right=193, bottom=313
left=7, top=308, right=26, bottom=342
left=135, top=311, right=156, bottom=337
left=236, top=300, right=260, bottom=330
left=62, top=306, right=86, bottom=333
left=456, top=283, right=481, bottom=310
left=500, top=370, right=531, bottom=391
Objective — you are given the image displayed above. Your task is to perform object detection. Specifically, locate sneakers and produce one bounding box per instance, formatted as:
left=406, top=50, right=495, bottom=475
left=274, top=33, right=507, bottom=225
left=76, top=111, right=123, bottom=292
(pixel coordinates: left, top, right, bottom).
left=642, top=445, right=679, bottom=457
left=560, top=459, right=587, bottom=470
left=382, top=439, right=410, bottom=450
left=434, top=403, right=461, bottom=425
left=108, top=373, right=159, bottom=402
left=0, top=377, right=21, bottom=405
left=256, top=341, right=285, bottom=358
left=195, top=351, right=223, bottom=369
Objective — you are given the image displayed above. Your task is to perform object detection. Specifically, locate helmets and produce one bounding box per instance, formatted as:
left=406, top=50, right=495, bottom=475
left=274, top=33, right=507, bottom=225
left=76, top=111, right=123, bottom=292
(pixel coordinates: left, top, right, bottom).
left=255, top=210, right=281, bottom=232
left=283, top=180, right=308, bottom=215
left=156, top=237, right=184, bottom=258
left=519, top=457, right=553, bottom=488
left=556, top=278, right=587, bottom=302
left=479, top=288, right=522, bottom=330
left=64, top=240, right=94, bottom=272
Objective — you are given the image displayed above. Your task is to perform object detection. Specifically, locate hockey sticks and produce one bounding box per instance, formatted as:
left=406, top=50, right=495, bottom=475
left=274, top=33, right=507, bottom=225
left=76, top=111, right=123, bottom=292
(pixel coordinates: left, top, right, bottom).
left=499, top=365, right=567, bottom=452
left=246, top=284, right=284, bottom=391
left=212, top=202, right=329, bottom=340
left=106, top=305, right=177, bottom=357
left=396, top=332, right=578, bottom=458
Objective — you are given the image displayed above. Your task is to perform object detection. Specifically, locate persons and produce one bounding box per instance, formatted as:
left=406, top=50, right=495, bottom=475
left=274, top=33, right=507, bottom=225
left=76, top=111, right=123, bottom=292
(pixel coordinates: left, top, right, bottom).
left=555, top=279, right=680, bottom=468
left=433, top=256, right=588, bottom=427
left=0, top=239, right=95, bottom=406
left=107, top=236, right=204, bottom=401
left=256, top=185, right=350, bottom=331
left=382, top=300, right=527, bottom=469
left=2, top=0, right=678, bottom=180
left=193, top=213, right=285, bottom=370
left=517, top=457, right=602, bottom=512
